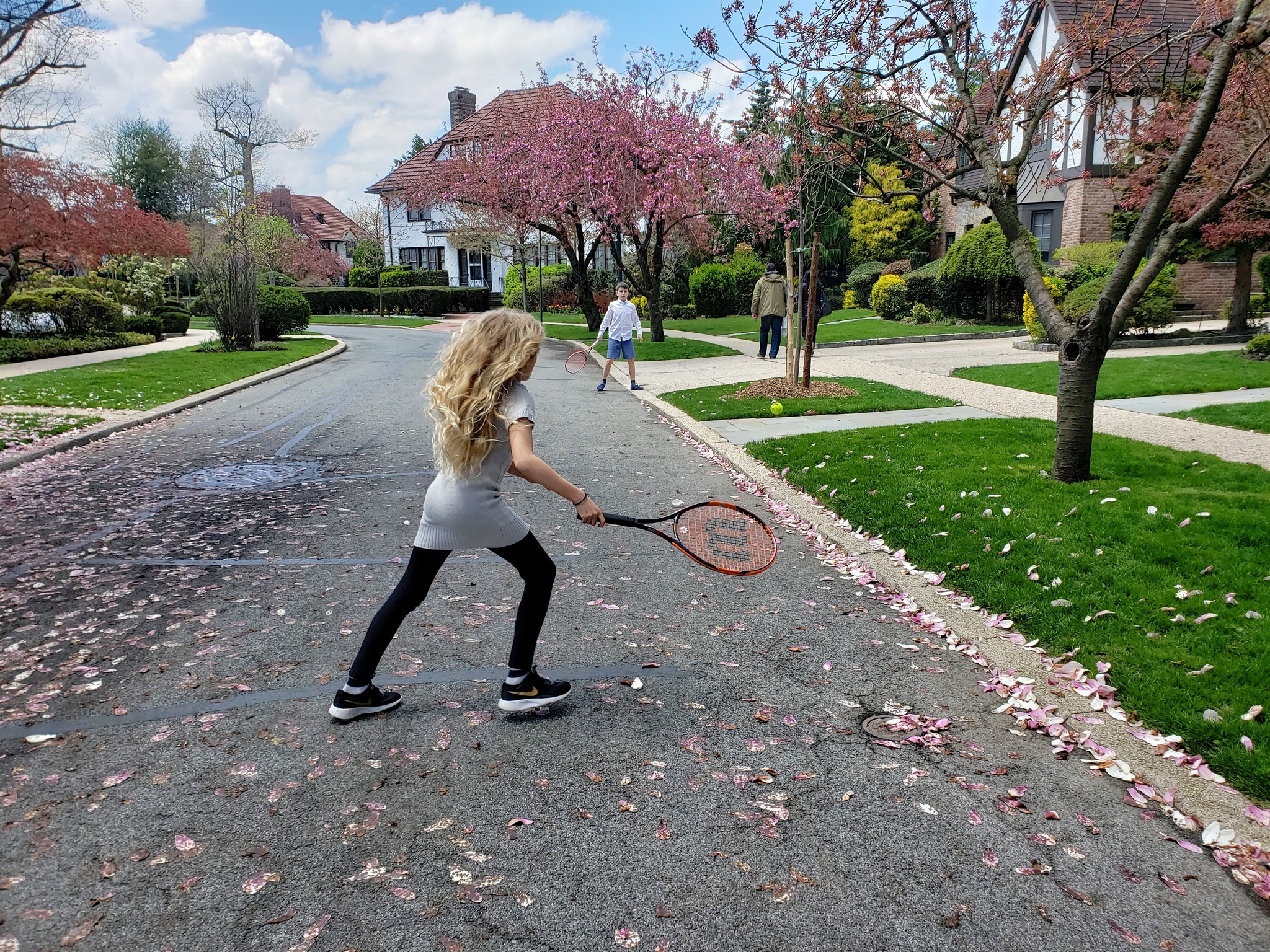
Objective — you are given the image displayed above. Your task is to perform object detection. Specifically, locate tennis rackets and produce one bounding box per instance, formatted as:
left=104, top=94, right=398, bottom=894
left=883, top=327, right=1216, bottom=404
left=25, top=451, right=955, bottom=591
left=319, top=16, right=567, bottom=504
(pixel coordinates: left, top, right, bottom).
left=579, top=498, right=776, bottom=576
left=565, top=338, right=602, bottom=373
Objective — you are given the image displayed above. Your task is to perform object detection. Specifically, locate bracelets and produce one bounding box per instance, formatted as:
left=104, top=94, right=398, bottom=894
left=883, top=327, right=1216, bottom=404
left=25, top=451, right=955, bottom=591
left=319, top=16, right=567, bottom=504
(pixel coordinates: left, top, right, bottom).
left=573, top=493, right=586, bottom=505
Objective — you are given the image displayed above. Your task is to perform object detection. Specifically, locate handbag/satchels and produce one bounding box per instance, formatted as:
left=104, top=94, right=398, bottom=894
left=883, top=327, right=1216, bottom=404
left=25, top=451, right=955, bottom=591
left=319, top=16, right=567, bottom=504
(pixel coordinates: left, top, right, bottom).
left=820, top=287, right=832, bottom=317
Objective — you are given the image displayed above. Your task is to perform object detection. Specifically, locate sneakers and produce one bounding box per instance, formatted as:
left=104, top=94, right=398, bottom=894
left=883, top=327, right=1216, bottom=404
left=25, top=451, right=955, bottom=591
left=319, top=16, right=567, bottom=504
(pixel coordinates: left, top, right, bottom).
left=757, top=353, right=765, bottom=357
left=770, top=356, right=776, bottom=359
left=596, top=381, right=606, bottom=391
left=498, top=665, right=571, bottom=711
left=328, top=673, right=402, bottom=721
left=630, top=381, right=644, bottom=390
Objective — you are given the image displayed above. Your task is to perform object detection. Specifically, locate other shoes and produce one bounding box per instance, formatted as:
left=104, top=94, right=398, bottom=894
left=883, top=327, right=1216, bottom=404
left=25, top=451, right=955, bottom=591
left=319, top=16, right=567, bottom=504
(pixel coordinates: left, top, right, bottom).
left=811, top=354, right=813, bottom=357
left=811, top=342, right=815, bottom=354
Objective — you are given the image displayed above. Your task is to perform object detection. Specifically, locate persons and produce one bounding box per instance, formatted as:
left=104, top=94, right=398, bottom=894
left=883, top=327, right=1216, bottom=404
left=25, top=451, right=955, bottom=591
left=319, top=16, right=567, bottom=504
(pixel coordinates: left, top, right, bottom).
left=795, top=270, right=825, bottom=358
left=326, top=305, right=605, bottom=721
left=751, top=263, right=787, bottom=359
left=596, top=282, right=644, bottom=391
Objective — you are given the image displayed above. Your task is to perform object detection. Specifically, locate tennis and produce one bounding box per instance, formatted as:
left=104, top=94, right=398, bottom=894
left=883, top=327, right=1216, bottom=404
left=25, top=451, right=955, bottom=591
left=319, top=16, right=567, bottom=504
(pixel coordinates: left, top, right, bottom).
left=771, top=402, right=782, bottom=415
